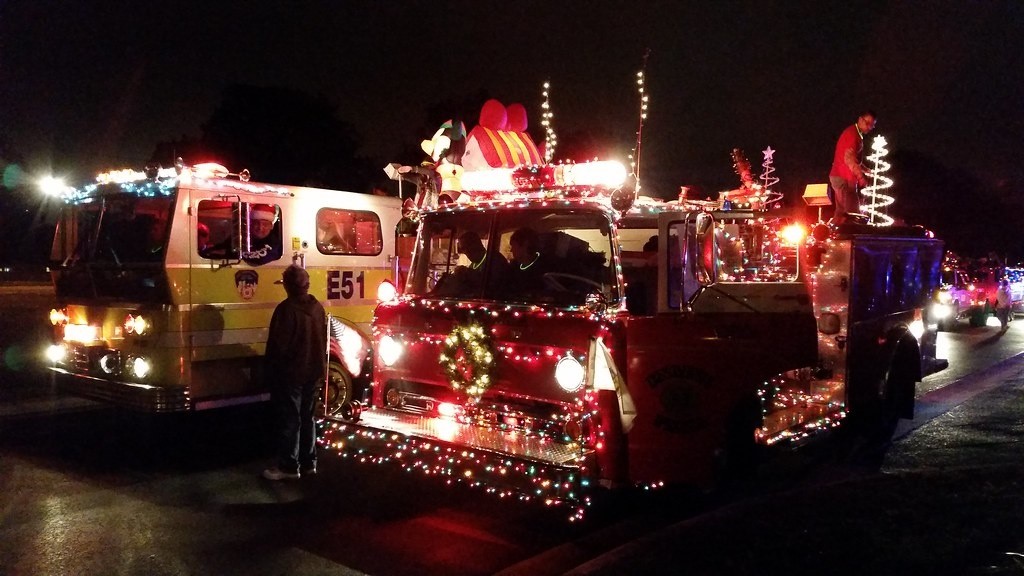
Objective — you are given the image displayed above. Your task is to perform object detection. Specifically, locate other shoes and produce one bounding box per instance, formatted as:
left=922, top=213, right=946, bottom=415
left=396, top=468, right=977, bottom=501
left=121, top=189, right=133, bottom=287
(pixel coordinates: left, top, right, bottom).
left=1001, top=325, right=1009, bottom=333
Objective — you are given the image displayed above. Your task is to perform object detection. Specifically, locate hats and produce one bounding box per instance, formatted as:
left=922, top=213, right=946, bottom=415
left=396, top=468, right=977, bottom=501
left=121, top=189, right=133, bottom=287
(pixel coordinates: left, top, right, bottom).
left=249, top=204, right=276, bottom=221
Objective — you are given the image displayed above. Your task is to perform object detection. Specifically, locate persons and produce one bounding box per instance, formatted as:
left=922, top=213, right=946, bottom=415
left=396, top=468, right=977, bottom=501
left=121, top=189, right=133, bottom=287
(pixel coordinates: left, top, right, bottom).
left=144, top=218, right=166, bottom=260
left=196, top=204, right=279, bottom=261
left=266, top=266, right=330, bottom=481
left=996, top=280, right=1013, bottom=333
left=829, top=111, right=876, bottom=223
left=452, top=229, right=558, bottom=304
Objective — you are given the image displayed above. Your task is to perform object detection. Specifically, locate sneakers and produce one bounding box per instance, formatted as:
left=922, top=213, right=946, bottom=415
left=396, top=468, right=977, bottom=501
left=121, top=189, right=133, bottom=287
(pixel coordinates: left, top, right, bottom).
left=262, top=466, right=301, bottom=481
left=304, top=467, right=319, bottom=477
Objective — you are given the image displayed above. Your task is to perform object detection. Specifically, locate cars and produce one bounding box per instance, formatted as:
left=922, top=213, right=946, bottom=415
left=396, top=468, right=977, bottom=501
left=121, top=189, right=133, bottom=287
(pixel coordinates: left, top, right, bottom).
left=41, top=152, right=417, bottom=423
left=347, top=159, right=1024, bottom=515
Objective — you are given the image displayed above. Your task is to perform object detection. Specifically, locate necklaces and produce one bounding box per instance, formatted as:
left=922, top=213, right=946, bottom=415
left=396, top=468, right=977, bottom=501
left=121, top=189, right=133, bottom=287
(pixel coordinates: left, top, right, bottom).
left=855, top=124, right=863, bottom=140
left=520, top=252, right=540, bottom=270
left=151, top=246, right=163, bottom=253
left=473, top=253, right=487, bottom=270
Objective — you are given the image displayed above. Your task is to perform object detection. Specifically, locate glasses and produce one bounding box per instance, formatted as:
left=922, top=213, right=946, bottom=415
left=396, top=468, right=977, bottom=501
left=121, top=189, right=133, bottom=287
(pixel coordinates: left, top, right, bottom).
left=862, top=117, right=875, bottom=128
